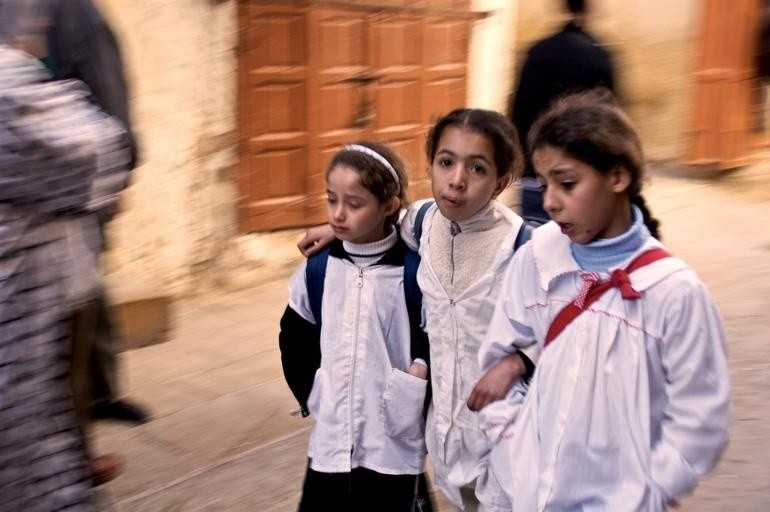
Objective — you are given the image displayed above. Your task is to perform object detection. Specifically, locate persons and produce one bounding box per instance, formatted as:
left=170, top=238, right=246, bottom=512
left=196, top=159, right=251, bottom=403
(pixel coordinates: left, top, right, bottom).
left=296, top=102, right=541, bottom=512
left=0, top=0, right=154, bottom=510
left=276, top=133, right=433, bottom=512
left=475, top=84, right=734, bottom=512
left=503, top=0, right=616, bottom=229
